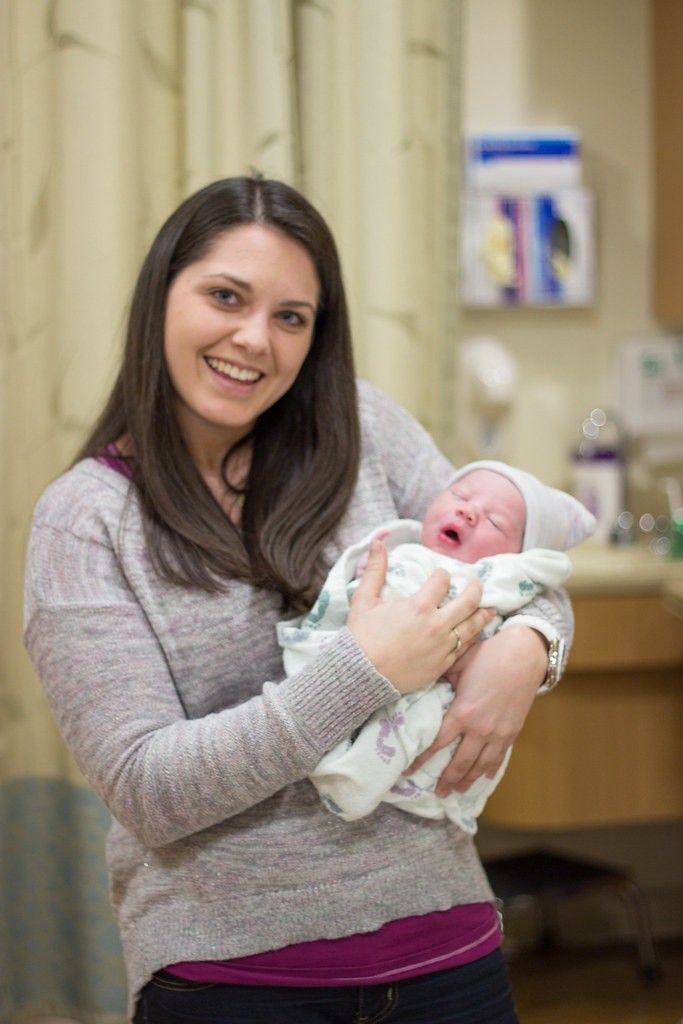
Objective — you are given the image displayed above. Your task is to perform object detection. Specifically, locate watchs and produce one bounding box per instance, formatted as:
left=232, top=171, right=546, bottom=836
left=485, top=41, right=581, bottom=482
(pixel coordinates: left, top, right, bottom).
left=504, top=614, right=567, bottom=695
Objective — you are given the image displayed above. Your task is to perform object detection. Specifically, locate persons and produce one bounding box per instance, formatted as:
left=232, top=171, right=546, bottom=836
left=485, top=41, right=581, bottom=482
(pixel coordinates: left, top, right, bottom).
left=23, top=177, right=576, bottom=1024
left=278, top=459, right=598, bottom=835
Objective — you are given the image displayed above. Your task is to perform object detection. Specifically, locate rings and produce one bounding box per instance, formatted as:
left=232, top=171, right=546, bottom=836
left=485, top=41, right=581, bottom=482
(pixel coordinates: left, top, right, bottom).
left=452, top=629, right=462, bottom=653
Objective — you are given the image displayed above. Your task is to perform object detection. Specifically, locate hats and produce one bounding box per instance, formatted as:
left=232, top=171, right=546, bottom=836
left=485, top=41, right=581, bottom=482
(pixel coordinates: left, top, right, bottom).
left=444, top=460, right=598, bottom=555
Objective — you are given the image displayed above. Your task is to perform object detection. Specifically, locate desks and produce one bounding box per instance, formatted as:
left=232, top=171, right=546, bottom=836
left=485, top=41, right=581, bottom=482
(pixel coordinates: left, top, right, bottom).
left=473, top=587, right=683, bottom=830
